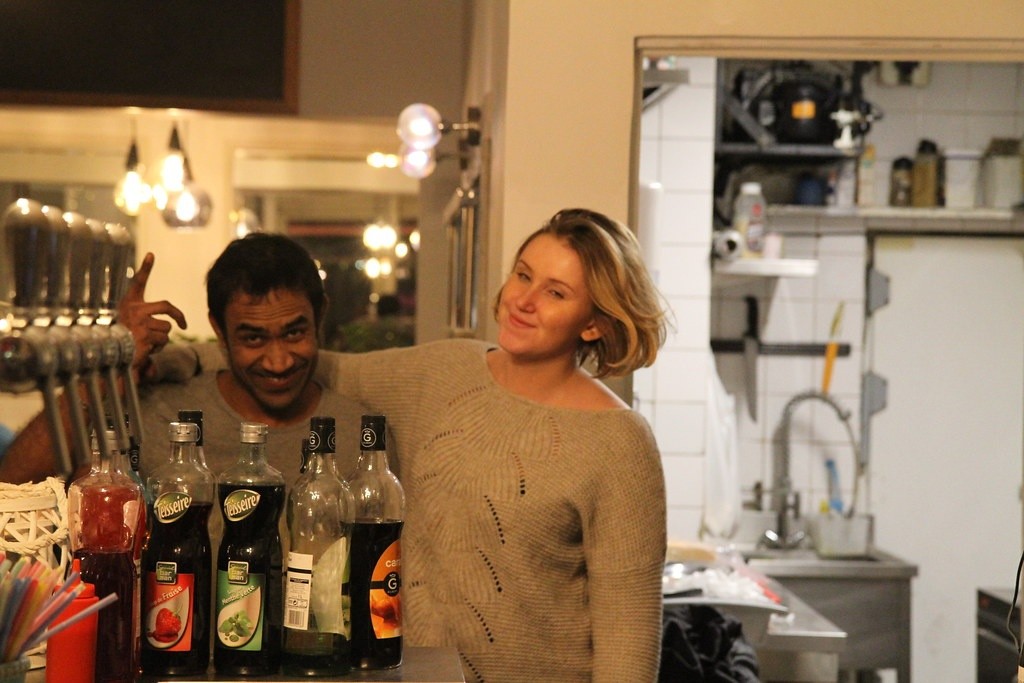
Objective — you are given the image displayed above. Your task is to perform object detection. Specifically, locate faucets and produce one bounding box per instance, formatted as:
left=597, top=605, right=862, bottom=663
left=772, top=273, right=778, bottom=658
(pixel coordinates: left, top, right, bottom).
left=766, top=387, right=851, bottom=549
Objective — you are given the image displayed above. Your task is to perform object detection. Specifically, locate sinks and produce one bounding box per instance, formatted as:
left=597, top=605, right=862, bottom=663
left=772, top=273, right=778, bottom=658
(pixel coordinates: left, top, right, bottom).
left=740, top=548, right=920, bottom=665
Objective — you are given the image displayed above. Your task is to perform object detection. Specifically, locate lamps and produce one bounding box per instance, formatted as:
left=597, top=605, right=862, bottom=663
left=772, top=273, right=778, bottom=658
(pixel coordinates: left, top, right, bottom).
left=396, top=103, right=483, bottom=176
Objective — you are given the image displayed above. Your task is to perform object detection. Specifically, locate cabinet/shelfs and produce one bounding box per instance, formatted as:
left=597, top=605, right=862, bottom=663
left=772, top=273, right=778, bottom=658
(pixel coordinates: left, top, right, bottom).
left=713, top=138, right=865, bottom=234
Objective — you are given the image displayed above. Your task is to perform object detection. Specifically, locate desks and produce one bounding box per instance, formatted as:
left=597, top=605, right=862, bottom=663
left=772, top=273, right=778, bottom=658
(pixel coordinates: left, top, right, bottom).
left=144, top=645, right=467, bottom=683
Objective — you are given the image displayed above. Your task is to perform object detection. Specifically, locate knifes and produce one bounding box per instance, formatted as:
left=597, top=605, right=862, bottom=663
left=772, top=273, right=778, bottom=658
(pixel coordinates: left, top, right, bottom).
left=743, top=295, right=761, bottom=419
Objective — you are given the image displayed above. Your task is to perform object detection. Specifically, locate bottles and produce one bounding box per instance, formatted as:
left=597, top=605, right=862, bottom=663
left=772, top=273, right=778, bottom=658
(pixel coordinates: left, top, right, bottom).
left=734, top=183, right=766, bottom=258
left=282, top=413, right=406, bottom=676
left=216, top=421, right=285, bottom=677
left=46, top=425, right=148, bottom=683
left=142, top=410, right=216, bottom=677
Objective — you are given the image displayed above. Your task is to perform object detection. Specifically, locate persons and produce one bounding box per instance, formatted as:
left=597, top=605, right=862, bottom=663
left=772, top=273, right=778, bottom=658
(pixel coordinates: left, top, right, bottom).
left=148, top=207, right=667, bottom=683
left=0, top=231, right=400, bottom=682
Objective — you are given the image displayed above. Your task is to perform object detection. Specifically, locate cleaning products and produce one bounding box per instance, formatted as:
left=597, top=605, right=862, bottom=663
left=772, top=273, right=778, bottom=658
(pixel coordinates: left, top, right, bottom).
left=731, top=182, right=770, bottom=261
